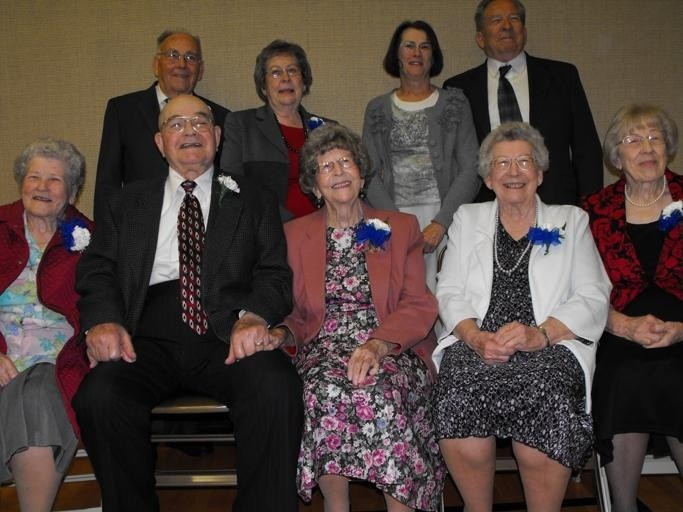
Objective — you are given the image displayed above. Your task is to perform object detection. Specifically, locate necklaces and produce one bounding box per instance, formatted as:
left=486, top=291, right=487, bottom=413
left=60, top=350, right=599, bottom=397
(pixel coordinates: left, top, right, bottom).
left=278, top=112, right=308, bottom=153
left=621, top=173, right=665, bottom=206
left=494, top=194, right=537, bottom=274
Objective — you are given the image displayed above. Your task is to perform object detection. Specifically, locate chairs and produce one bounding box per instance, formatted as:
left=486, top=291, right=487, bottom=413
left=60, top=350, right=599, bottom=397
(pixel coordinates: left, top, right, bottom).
left=439, top=436, right=611, bottom=511
left=150, top=392, right=238, bottom=486
left=52, top=444, right=101, bottom=512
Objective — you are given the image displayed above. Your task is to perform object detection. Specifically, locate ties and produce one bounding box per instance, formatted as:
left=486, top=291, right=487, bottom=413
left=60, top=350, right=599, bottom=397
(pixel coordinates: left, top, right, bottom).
left=177, top=180, right=211, bottom=336
left=497, top=65, right=523, bottom=125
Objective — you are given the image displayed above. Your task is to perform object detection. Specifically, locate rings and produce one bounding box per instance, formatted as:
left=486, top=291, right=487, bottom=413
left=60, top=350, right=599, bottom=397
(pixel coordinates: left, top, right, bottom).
left=254, top=342, right=261, bottom=347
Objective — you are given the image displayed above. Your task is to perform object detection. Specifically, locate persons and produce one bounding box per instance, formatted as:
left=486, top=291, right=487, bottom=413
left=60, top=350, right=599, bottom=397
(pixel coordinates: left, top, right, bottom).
left=264, top=123, right=437, bottom=511
left=220, top=41, right=343, bottom=225
left=436, top=122, right=611, bottom=512
left=72, top=94, right=301, bottom=511
left=362, top=22, right=483, bottom=293
left=93, top=30, right=233, bottom=223
left=443, top=0, right=602, bottom=205
left=0, top=141, right=135, bottom=512
left=577, top=105, right=682, bottom=511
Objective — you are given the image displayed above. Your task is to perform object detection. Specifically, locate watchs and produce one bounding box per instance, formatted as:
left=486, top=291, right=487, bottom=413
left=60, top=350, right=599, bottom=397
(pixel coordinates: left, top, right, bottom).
left=534, top=325, right=551, bottom=350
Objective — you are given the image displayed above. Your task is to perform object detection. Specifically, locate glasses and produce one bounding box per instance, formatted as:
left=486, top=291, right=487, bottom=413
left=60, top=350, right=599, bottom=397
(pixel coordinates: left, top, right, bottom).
left=265, top=66, right=300, bottom=78
left=157, top=52, right=200, bottom=65
left=159, top=116, right=215, bottom=132
left=315, top=156, right=354, bottom=173
left=615, top=131, right=669, bottom=145
left=489, top=155, right=538, bottom=170
